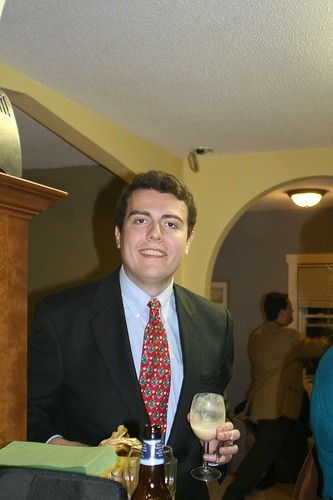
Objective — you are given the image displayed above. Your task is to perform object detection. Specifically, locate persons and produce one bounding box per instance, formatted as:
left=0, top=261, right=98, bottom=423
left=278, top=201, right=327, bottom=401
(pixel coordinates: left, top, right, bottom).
left=237, top=292, right=333, bottom=492
left=310, top=344, right=333, bottom=500
left=27, top=169, right=240, bottom=500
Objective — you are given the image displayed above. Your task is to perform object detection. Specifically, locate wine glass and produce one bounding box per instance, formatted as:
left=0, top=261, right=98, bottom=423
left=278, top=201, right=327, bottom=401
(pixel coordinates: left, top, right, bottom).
left=189, top=392, right=226, bottom=481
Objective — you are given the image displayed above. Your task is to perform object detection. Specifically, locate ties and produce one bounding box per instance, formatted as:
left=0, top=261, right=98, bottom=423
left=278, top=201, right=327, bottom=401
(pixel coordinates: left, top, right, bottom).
left=139, top=298, right=171, bottom=445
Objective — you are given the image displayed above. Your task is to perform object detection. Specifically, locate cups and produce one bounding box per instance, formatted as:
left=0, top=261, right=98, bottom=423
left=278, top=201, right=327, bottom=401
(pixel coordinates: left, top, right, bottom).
left=108, top=447, right=183, bottom=500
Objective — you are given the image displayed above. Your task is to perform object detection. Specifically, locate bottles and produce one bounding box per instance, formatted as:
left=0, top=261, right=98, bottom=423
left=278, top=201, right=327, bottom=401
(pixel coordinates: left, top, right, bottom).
left=131, top=424, right=172, bottom=500
